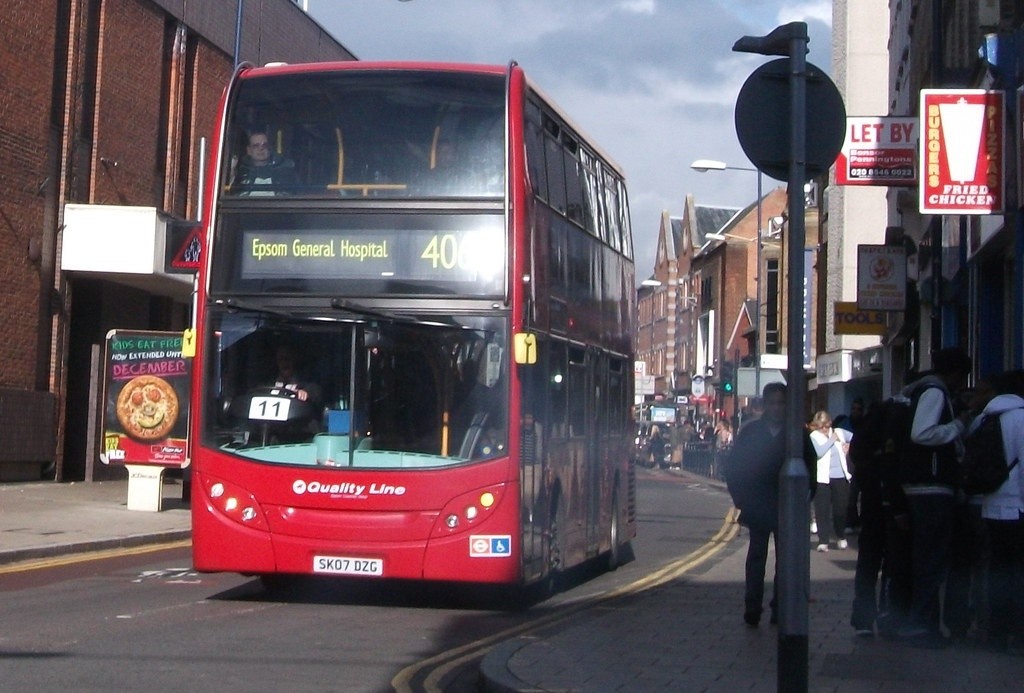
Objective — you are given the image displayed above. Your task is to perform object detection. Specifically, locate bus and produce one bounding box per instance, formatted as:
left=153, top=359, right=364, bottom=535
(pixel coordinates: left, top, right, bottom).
left=180, top=59, right=639, bottom=599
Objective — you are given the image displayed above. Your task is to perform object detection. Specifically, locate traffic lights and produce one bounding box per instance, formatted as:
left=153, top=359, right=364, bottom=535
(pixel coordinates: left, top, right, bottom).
left=721, top=373, right=735, bottom=396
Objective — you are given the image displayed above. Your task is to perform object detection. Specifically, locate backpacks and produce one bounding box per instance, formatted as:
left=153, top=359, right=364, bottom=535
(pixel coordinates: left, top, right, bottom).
left=964, top=406, right=1020, bottom=497
left=872, top=381, right=947, bottom=486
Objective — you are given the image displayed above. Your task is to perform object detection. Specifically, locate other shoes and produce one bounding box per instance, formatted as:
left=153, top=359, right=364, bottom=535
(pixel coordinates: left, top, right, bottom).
left=769, top=605, right=780, bottom=624
left=817, top=543, right=829, bottom=552
left=854, top=621, right=871, bottom=635
left=744, top=606, right=762, bottom=623
left=837, top=539, right=848, bottom=548
left=879, top=626, right=899, bottom=640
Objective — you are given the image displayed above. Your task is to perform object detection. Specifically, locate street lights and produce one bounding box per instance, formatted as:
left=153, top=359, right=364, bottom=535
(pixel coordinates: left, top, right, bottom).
left=689, top=155, right=765, bottom=422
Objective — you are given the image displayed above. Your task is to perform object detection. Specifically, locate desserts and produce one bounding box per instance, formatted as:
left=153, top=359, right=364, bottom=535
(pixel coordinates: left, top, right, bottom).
left=116, top=375, right=178, bottom=441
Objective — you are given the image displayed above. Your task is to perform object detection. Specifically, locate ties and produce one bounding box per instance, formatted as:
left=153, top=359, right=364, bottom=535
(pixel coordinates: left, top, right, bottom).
left=278, top=380, right=289, bottom=396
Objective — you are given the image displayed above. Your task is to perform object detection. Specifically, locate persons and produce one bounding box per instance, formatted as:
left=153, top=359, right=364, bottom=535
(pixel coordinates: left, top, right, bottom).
left=239, top=342, right=324, bottom=448
left=647, top=417, right=741, bottom=471
left=723, top=380, right=819, bottom=625
left=548, top=410, right=636, bottom=529
left=230, top=133, right=304, bottom=200
left=801, top=395, right=865, bottom=534
left=809, top=411, right=857, bottom=551
left=968, top=370, right=1024, bottom=648
left=521, top=409, right=551, bottom=523
left=419, top=141, right=476, bottom=196
left=844, top=343, right=980, bottom=643
